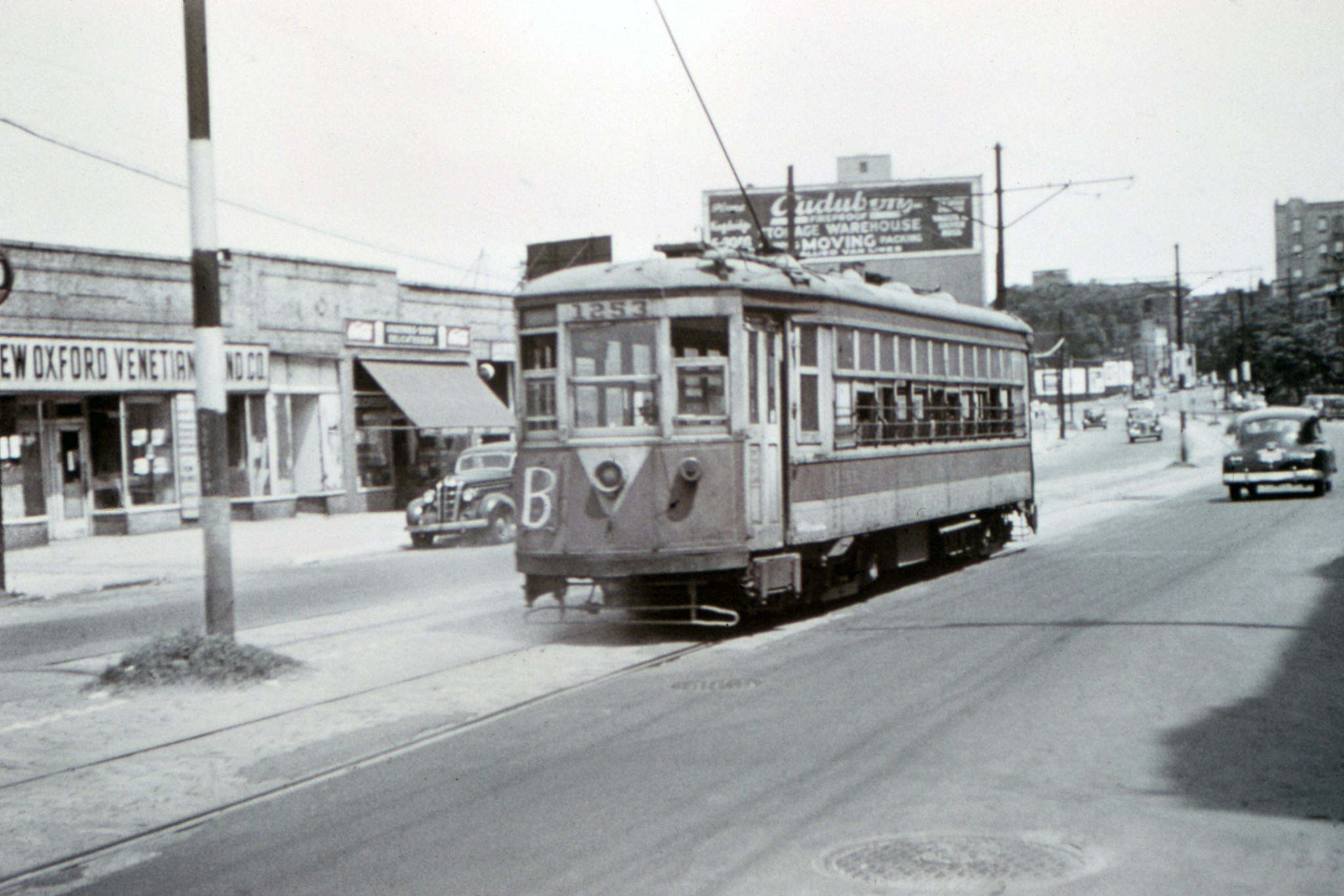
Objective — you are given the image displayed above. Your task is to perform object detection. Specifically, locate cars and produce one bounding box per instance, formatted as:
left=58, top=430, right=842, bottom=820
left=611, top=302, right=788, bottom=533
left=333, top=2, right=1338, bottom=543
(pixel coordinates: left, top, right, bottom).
left=1082, top=404, right=1107, bottom=430
left=403, top=442, right=517, bottom=544
left=1127, top=376, right=1163, bottom=444
left=1222, top=393, right=1344, bottom=501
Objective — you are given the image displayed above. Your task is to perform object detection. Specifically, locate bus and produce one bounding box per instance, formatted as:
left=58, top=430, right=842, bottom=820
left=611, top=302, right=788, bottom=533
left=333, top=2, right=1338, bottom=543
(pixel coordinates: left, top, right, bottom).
left=513, top=1, right=1039, bottom=628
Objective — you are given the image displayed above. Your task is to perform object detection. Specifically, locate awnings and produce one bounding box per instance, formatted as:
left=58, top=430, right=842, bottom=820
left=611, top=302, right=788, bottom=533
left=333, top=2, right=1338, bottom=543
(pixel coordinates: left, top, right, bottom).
left=357, top=355, right=517, bottom=436
